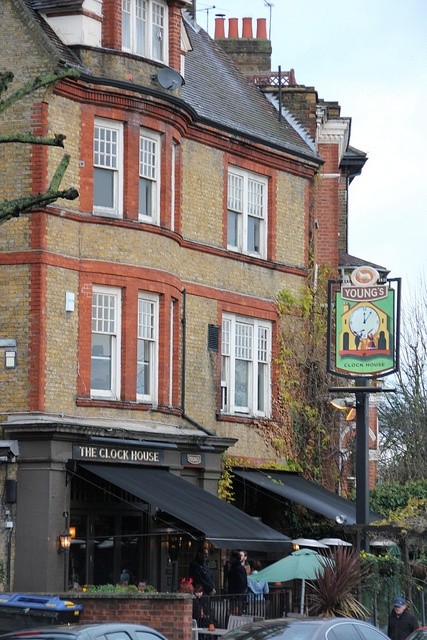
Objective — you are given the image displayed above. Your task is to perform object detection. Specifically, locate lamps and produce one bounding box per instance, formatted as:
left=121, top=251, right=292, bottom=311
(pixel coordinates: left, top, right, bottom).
left=57, top=529, right=71, bottom=553
left=69, top=527, right=76, bottom=538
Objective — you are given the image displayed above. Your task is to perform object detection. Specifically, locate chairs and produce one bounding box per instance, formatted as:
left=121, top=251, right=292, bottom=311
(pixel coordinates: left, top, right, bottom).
left=227, top=616, right=254, bottom=633
left=242, top=615, right=264, bottom=624
left=283, top=612, right=310, bottom=620
left=192, top=619, right=198, bottom=640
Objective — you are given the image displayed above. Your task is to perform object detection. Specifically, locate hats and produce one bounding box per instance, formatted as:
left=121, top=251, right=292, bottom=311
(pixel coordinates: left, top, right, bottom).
left=394, top=598, right=406, bottom=607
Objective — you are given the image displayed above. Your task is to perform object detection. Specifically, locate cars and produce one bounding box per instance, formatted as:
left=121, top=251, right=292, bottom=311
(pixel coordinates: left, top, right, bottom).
left=218, top=616, right=391, bottom=640
left=0, top=623, right=168, bottom=639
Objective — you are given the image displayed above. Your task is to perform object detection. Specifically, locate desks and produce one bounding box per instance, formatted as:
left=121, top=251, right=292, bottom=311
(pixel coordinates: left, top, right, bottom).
left=194, top=628, right=228, bottom=640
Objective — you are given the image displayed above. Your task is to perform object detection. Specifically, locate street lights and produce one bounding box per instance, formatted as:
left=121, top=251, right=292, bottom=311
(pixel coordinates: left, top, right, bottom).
left=330, top=398, right=369, bottom=554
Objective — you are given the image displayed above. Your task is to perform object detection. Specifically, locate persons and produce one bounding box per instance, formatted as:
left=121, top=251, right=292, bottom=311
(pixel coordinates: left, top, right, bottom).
left=114, top=520, right=305, bottom=638
left=387, top=598, right=421, bottom=640
